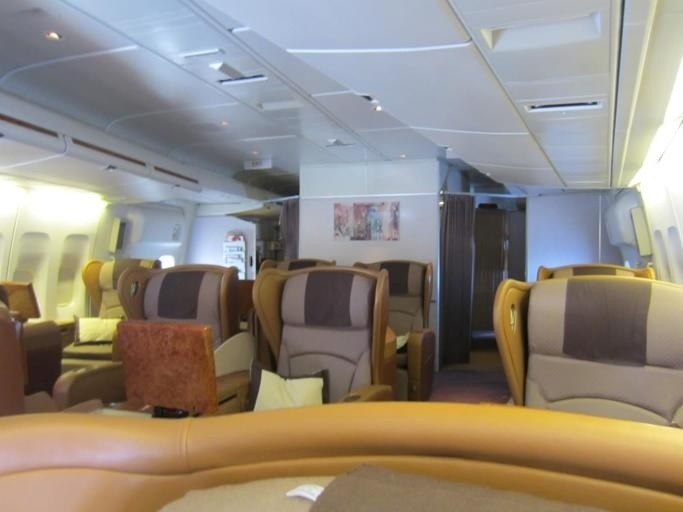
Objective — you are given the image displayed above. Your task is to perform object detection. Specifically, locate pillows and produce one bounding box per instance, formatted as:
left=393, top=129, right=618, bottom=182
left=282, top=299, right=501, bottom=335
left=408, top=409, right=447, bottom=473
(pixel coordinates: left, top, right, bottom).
left=396, top=330, right=407, bottom=351
left=242, top=364, right=328, bottom=412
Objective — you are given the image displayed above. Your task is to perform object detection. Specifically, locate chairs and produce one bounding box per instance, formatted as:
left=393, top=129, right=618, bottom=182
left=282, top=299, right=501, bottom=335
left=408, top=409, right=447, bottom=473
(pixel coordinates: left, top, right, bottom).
left=2, top=260, right=160, bottom=419
left=183, top=265, right=393, bottom=412
left=2, top=410, right=682, bottom=512
left=493, top=278, right=682, bottom=441
left=353, top=260, right=439, bottom=403
left=253, top=259, right=336, bottom=361
left=48, top=263, right=236, bottom=410
left=537, top=265, right=654, bottom=279
